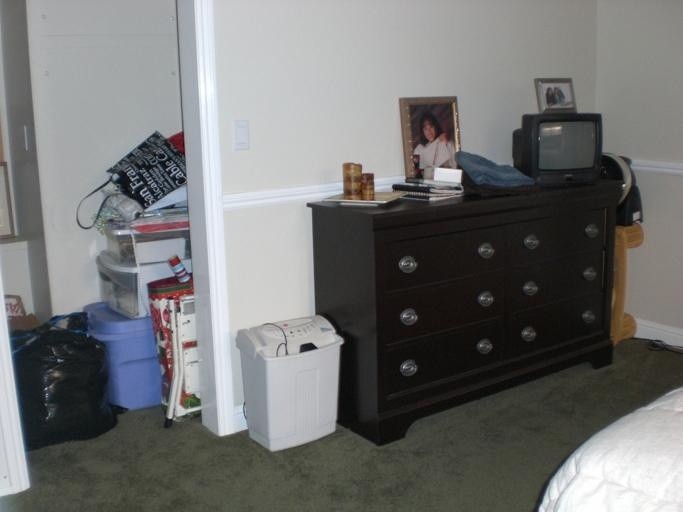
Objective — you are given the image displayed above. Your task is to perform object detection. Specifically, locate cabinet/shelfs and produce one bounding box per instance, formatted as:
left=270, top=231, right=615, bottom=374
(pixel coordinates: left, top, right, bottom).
left=308, top=179, right=627, bottom=444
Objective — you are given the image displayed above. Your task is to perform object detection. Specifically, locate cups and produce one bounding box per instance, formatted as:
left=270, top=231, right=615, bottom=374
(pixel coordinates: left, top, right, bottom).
left=362, top=174, right=374, bottom=201
left=343, top=163, right=363, bottom=201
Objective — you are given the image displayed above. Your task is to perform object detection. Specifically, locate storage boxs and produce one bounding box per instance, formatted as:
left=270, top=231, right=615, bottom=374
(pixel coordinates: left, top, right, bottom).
left=94, top=248, right=194, bottom=321
left=103, top=210, right=194, bottom=268
left=80, top=302, right=163, bottom=409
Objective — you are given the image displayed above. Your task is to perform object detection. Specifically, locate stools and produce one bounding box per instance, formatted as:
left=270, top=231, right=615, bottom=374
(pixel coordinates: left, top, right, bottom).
left=146, top=276, right=202, bottom=428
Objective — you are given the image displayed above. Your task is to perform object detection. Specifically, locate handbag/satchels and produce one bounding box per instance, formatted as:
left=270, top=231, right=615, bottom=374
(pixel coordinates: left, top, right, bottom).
left=75, top=131, right=186, bottom=229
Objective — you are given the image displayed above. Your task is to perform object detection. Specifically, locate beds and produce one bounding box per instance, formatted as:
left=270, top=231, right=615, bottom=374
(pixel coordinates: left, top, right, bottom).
left=533, top=387, right=682, bottom=509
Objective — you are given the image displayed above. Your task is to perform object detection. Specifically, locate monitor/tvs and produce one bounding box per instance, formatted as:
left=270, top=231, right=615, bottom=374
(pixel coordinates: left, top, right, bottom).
left=513, top=112, right=603, bottom=188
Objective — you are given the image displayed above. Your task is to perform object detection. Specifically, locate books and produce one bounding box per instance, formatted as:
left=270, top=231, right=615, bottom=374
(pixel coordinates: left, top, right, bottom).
left=390, top=178, right=464, bottom=203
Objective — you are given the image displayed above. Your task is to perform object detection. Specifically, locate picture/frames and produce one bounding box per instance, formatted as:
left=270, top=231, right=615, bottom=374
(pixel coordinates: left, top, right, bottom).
left=398, top=95, right=465, bottom=184
left=534, top=75, right=579, bottom=115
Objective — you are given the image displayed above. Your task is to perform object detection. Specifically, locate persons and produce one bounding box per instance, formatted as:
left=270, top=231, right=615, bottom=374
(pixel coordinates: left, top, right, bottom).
left=554, top=88, right=565, bottom=103
left=544, top=87, right=555, bottom=105
left=412, top=112, right=455, bottom=176
